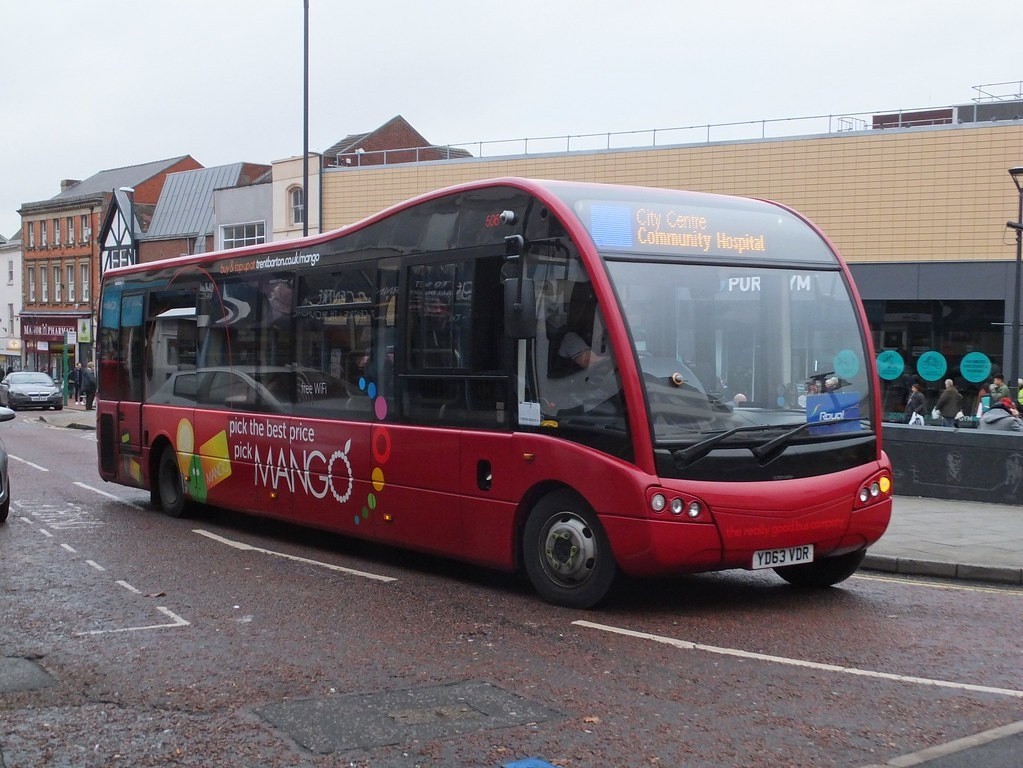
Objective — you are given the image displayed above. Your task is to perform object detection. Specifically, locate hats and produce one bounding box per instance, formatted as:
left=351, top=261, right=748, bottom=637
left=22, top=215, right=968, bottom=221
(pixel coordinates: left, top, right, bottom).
left=999, top=398, right=1013, bottom=408
left=87, top=362, right=94, bottom=367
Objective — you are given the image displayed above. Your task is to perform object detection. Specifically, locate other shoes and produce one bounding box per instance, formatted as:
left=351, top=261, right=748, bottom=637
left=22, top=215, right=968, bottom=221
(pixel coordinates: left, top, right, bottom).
left=75, top=402, right=79, bottom=406
left=80, top=402, right=84, bottom=405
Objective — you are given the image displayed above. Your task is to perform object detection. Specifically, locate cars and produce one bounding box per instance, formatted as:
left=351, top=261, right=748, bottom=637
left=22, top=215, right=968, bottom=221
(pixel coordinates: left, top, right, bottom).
left=0, top=403, right=18, bottom=522
left=146, top=361, right=372, bottom=419
left=0, top=372, right=65, bottom=410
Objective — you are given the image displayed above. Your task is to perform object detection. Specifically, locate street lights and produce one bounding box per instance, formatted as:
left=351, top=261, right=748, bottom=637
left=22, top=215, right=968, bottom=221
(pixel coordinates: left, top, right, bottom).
left=1009, top=167, right=1023, bottom=407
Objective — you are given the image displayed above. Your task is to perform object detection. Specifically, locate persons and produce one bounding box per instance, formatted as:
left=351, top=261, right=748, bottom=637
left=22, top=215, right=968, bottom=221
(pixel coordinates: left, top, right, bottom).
left=6, top=367, right=14, bottom=375
left=808, top=377, right=839, bottom=395
left=977, top=375, right=1023, bottom=432
left=935, top=379, right=959, bottom=428
left=724, top=393, right=747, bottom=406
left=68, top=362, right=97, bottom=410
left=547, top=298, right=612, bottom=375
left=905, top=384, right=929, bottom=423
left=0, top=365, right=5, bottom=383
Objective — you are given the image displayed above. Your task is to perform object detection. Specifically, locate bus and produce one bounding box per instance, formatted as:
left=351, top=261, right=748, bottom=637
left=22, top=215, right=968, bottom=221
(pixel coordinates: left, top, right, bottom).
left=94, top=176, right=894, bottom=611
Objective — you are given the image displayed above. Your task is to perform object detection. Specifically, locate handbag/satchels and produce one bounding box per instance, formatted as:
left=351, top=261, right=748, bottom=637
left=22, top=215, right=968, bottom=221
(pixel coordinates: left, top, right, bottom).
left=954, top=411, right=964, bottom=420
left=931, top=409, right=941, bottom=419
left=909, top=412, right=925, bottom=426
